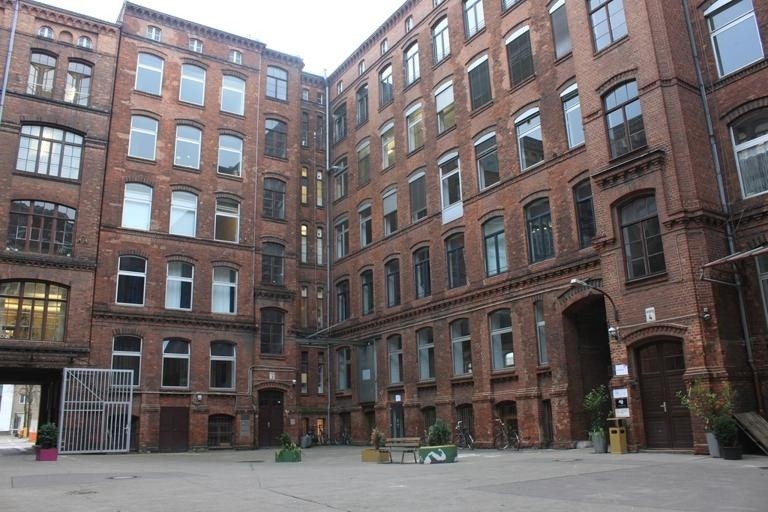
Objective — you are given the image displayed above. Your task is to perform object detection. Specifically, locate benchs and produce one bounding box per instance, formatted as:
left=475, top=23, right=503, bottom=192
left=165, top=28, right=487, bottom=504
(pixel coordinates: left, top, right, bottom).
left=378, top=436, right=422, bottom=464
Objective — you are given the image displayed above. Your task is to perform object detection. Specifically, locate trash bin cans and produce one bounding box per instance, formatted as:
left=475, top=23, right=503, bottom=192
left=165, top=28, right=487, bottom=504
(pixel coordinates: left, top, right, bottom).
left=607, top=417, right=628, bottom=455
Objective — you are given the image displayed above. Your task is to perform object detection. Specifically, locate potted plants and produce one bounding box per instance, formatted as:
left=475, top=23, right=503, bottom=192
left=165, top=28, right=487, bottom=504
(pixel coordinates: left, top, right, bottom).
left=34, top=420, right=60, bottom=461
left=361, top=426, right=389, bottom=462
left=582, top=383, right=611, bottom=453
left=419, top=416, right=458, bottom=464
left=675, top=373, right=744, bottom=460
left=275, top=432, right=303, bottom=462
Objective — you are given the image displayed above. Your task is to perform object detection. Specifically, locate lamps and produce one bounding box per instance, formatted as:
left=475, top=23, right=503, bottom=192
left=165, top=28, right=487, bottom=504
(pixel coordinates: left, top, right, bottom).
left=570, top=278, right=619, bottom=322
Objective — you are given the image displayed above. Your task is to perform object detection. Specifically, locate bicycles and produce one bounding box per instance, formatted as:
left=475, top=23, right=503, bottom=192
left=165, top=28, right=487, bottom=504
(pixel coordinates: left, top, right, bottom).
left=490, top=416, right=521, bottom=452
left=306, top=424, right=353, bottom=445
left=453, top=420, right=475, bottom=450
